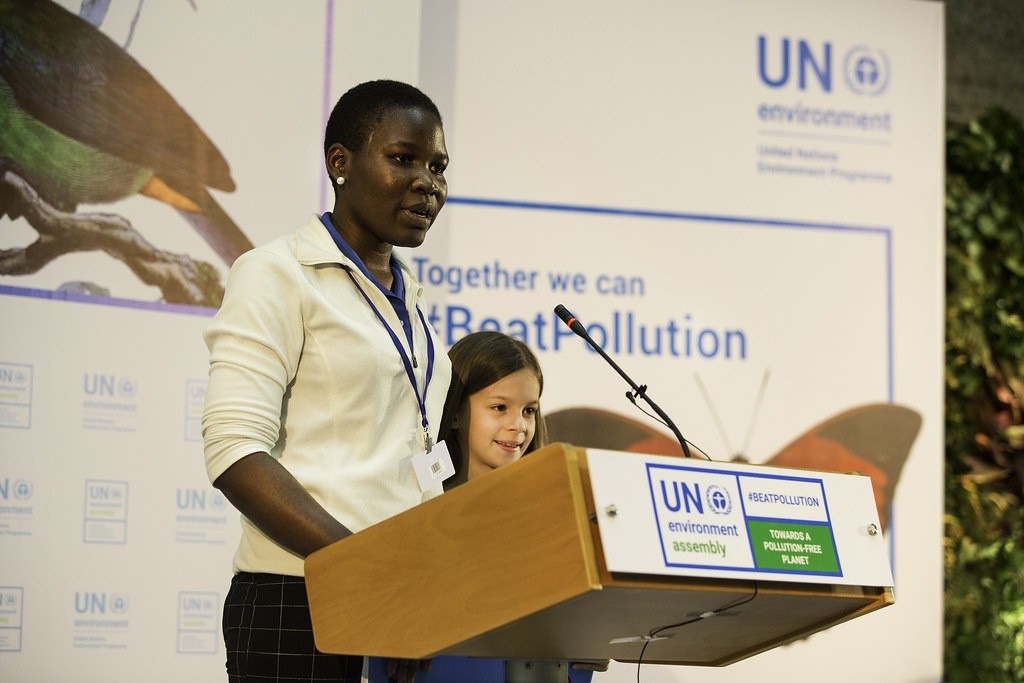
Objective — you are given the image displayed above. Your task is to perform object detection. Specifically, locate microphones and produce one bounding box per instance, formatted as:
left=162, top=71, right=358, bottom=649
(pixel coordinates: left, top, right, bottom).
left=554, top=304, right=691, bottom=458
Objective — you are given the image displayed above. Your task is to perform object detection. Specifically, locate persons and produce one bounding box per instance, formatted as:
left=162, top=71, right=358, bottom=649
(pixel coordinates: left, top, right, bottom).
left=200, top=79, right=452, bottom=683
left=368, top=330, right=593, bottom=683
